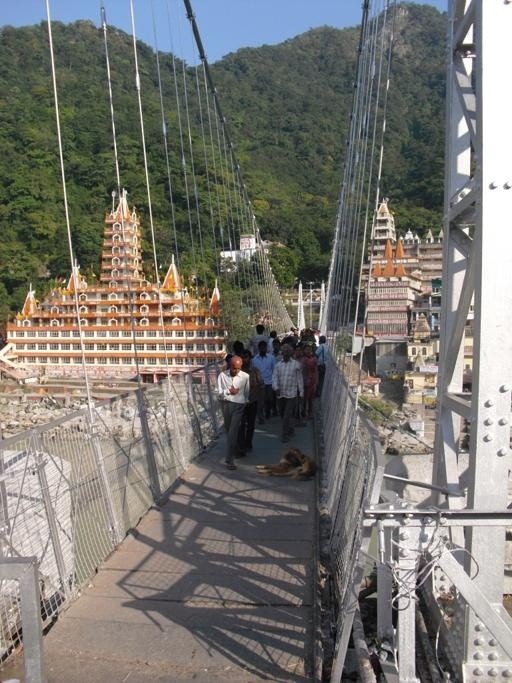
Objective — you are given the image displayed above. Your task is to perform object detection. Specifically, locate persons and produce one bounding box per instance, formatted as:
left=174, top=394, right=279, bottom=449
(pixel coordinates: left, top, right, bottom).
left=217, top=324, right=328, bottom=470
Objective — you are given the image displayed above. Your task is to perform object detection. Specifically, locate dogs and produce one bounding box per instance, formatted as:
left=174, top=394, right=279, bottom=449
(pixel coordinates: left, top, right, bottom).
left=256, top=447, right=317, bottom=481
